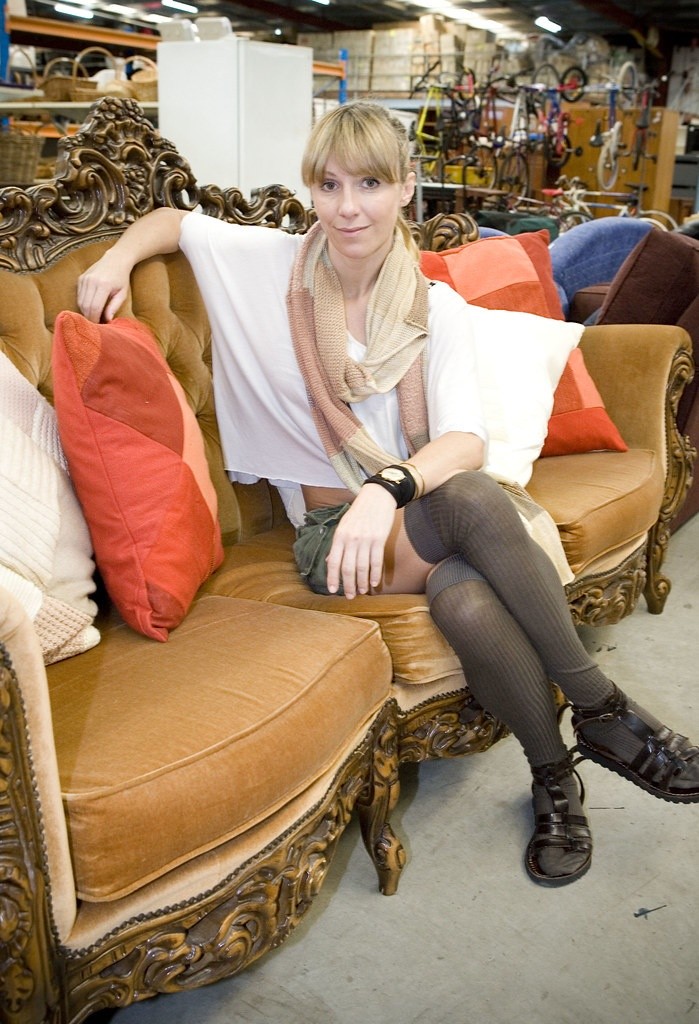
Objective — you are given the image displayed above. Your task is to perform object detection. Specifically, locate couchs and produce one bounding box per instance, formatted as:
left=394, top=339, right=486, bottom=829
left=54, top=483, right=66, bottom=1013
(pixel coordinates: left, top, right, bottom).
left=475, top=216, right=699, bottom=536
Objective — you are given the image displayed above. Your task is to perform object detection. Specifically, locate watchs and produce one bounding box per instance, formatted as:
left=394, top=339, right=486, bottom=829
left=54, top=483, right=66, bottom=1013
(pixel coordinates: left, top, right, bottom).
left=375, top=468, right=412, bottom=508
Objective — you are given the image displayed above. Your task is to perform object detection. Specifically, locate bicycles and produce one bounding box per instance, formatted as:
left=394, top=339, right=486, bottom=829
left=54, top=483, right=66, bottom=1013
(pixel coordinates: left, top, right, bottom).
left=406, top=58, right=682, bottom=240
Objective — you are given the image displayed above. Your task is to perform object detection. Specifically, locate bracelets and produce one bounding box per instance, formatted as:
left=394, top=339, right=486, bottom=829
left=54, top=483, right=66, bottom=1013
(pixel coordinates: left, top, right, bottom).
left=361, top=463, right=424, bottom=510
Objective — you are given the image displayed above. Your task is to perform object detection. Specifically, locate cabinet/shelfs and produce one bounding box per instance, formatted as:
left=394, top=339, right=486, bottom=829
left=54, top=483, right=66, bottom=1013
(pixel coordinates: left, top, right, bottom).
left=499, top=106, right=679, bottom=230
left=0, top=0, right=349, bottom=195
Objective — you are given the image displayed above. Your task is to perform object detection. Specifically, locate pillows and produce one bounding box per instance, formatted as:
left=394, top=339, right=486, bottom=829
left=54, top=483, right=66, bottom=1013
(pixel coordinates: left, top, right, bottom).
left=0, top=96, right=699, bottom=1024
left=0, top=349, right=102, bottom=667
left=51, top=309, right=225, bottom=642
left=415, top=229, right=629, bottom=458
left=464, top=304, right=585, bottom=487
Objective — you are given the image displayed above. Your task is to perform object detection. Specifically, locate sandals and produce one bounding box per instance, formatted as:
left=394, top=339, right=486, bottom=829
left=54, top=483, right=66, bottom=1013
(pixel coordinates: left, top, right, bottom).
left=556, top=679, right=699, bottom=805
left=524, top=744, right=594, bottom=888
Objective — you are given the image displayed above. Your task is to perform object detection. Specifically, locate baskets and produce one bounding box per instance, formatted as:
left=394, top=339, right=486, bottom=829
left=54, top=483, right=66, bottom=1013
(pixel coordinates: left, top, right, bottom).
left=69, top=47, right=118, bottom=102
left=118, top=55, right=157, bottom=101
left=37, top=57, right=98, bottom=101
left=0, top=125, right=40, bottom=186
left=34, top=121, right=68, bottom=178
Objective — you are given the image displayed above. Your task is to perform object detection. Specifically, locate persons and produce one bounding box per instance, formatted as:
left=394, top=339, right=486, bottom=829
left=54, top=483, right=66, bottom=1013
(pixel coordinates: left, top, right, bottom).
left=75, top=91, right=699, bottom=888
